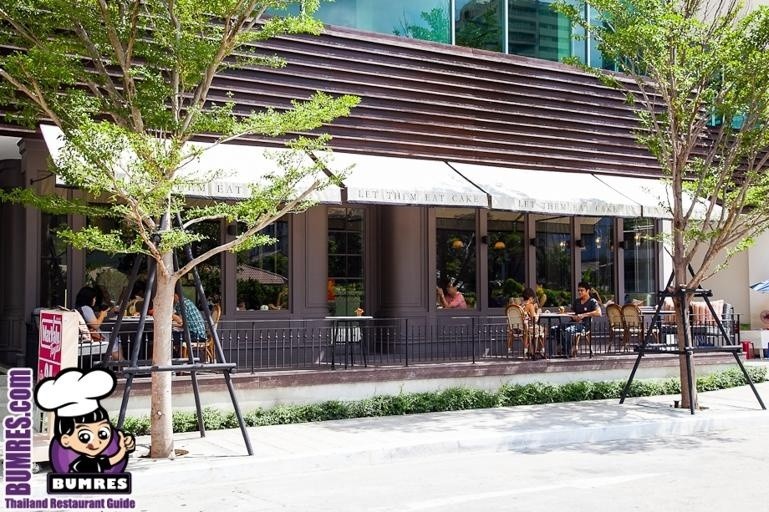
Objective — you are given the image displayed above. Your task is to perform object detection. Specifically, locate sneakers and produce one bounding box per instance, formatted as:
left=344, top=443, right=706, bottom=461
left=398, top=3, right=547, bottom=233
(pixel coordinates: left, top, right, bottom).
left=526, top=352, right=544, bottom=359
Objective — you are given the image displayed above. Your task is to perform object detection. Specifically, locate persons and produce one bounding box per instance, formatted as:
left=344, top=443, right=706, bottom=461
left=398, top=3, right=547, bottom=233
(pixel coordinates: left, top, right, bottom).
left=515, top=287, right=546, bottom=358
left=551, top=281, right=602, bottom=358
left=52, top=405, right=136, bottom=475
left=148, top=284, right=209, bottom=343
left=437, top=280, right=469, bottom=309
left=268, top=286, right=288, bottom=310
left=75, top=285, right=127, bottom=362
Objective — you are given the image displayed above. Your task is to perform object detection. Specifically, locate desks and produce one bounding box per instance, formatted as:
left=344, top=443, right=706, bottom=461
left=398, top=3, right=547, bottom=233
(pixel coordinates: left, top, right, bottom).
left=640, top=310, right=675, bottom=347
left=324, top=315, right=373, bottom=370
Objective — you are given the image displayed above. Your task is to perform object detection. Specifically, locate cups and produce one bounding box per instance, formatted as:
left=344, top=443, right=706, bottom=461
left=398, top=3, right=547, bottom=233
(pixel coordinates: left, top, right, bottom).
left=558, top=305, right=565, bottom=314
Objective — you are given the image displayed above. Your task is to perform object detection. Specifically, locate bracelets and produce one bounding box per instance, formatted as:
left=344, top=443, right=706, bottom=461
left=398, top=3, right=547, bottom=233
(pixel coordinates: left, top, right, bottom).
left=441, top=294, right=444, bottom=298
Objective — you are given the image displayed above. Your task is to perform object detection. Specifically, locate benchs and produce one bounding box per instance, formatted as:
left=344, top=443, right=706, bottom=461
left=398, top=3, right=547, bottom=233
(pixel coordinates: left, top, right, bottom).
left=661, top=303, right=732, bottom=345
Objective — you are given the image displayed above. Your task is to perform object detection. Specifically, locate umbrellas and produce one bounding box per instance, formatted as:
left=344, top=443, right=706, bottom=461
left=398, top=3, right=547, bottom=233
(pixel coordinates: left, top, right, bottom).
left=748, top=279, right=769, bottom=294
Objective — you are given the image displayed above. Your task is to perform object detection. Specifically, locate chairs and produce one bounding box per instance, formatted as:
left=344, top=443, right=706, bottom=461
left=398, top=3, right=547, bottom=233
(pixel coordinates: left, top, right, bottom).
left=503, top=304, right=593, bottom=358
left=607, top=304, right=660, bottom=352
left=58, top=303, right=222, bottom=363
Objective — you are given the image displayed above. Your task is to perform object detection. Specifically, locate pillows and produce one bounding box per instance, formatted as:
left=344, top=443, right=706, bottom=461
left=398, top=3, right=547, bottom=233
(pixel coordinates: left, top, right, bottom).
left=664, top=300, right=725, bottom=327
